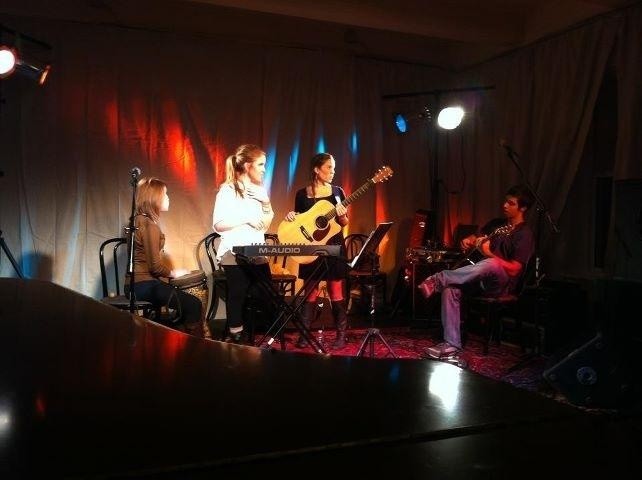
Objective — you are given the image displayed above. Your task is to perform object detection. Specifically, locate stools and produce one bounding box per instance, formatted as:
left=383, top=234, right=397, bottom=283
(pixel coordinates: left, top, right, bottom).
left=464, top=292, right=528, bottom=356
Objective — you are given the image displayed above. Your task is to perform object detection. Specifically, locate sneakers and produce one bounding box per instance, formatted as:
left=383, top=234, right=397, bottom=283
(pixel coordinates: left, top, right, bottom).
left=418, top=273, right=444, bottom=298
left=424, top=342, right=460, bottom=360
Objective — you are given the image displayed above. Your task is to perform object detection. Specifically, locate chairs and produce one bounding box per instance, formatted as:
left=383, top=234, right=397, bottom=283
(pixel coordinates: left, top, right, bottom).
left=341, top=231, right=388, bottom=323
left=97, top=237, right=160, bottom=325
left=204, top=231, right=297, bottom=337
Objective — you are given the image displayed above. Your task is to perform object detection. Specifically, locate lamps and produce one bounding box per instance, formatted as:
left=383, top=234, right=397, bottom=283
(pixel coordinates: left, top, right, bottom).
left=393, top=109, right=423, bottom=136
left=428, top=94, right=465, bottom=131
left=0, top=44, right=52, bottom=86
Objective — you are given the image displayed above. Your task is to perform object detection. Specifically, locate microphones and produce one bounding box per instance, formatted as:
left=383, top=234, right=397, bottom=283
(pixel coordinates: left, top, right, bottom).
left=128, top=167, right=141, bottom=180
left=499, top=137, right=520, bottom=156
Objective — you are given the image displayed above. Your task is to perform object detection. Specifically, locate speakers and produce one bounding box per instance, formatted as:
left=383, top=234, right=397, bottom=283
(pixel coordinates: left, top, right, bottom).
left=412, top=263, right=450, bottom=321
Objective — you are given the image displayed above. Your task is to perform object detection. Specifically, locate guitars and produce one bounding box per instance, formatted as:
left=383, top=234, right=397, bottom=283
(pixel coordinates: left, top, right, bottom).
left=449, top=224, right=514, bottom=269
left=277, top=166, right=394, bottom=265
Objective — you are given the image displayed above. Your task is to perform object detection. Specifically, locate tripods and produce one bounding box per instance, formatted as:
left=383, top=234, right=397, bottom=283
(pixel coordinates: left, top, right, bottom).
left=355, top=253, right=400, bottom=360
left=509, top=155, right=559, bottom=373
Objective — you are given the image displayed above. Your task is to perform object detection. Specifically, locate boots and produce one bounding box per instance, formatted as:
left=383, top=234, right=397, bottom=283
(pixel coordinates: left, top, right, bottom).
left=296, top=299, right=316, bottom=348
left=330, top=298, right=351, bottom=348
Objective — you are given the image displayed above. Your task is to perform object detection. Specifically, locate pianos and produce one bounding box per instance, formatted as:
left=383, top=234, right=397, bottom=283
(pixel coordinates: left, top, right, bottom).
left=232, top=242, right=342, bottom=257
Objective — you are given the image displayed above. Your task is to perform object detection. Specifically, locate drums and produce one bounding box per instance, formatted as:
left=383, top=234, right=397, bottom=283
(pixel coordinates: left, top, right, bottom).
left=170, top=269, right=213, bottom=341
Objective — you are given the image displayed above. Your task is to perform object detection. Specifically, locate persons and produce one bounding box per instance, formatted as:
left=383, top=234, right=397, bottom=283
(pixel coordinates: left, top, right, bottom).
left=284, top=153, right=350, bottom=350
left=125, top=175, right=204, bottom=341
left=211, top=142, right=280, bottom=348
left=417, top=185, right=536, bottom=361
